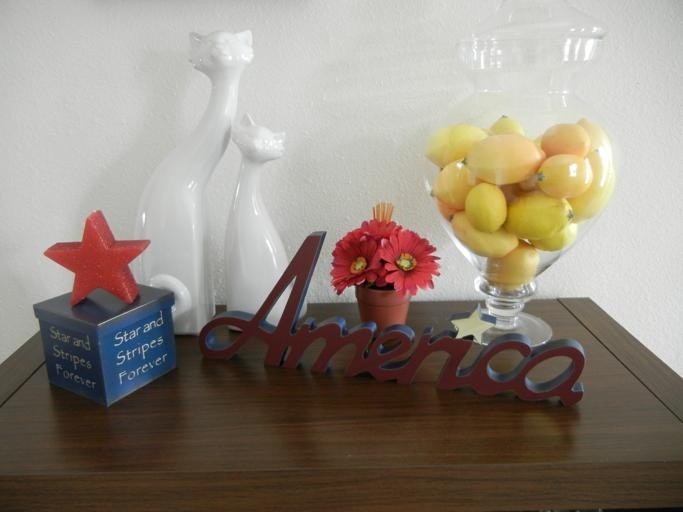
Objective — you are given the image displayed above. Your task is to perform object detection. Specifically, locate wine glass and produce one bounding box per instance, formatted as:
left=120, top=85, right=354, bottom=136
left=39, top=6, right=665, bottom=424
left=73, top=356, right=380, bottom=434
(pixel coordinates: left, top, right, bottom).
left=419, top=95, right=618, bottom=349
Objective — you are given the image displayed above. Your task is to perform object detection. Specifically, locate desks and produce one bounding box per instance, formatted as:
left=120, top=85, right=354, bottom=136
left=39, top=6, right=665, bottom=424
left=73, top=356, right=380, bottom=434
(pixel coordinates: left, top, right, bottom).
left=0, top=296, right=682, bottom=510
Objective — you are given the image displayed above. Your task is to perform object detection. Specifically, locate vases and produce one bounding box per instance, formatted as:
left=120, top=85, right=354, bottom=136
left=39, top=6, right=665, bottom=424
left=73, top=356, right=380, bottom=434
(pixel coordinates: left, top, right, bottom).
left=354, top=277, right=411, bottom=327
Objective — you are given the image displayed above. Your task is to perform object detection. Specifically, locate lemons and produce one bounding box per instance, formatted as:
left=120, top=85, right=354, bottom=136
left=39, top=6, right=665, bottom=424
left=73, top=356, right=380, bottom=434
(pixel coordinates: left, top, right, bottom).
left=425, top=115, right=615, bottom=292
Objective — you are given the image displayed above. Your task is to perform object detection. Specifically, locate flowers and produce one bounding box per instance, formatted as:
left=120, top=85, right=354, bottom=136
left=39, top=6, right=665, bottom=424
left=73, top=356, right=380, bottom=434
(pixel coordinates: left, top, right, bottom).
left=328, top=201, right=441, bottom=298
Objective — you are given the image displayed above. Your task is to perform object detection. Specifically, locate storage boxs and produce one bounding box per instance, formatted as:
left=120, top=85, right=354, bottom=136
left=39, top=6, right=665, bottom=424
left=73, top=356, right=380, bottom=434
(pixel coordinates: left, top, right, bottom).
left=30, top=283, right=180, bottom=409
